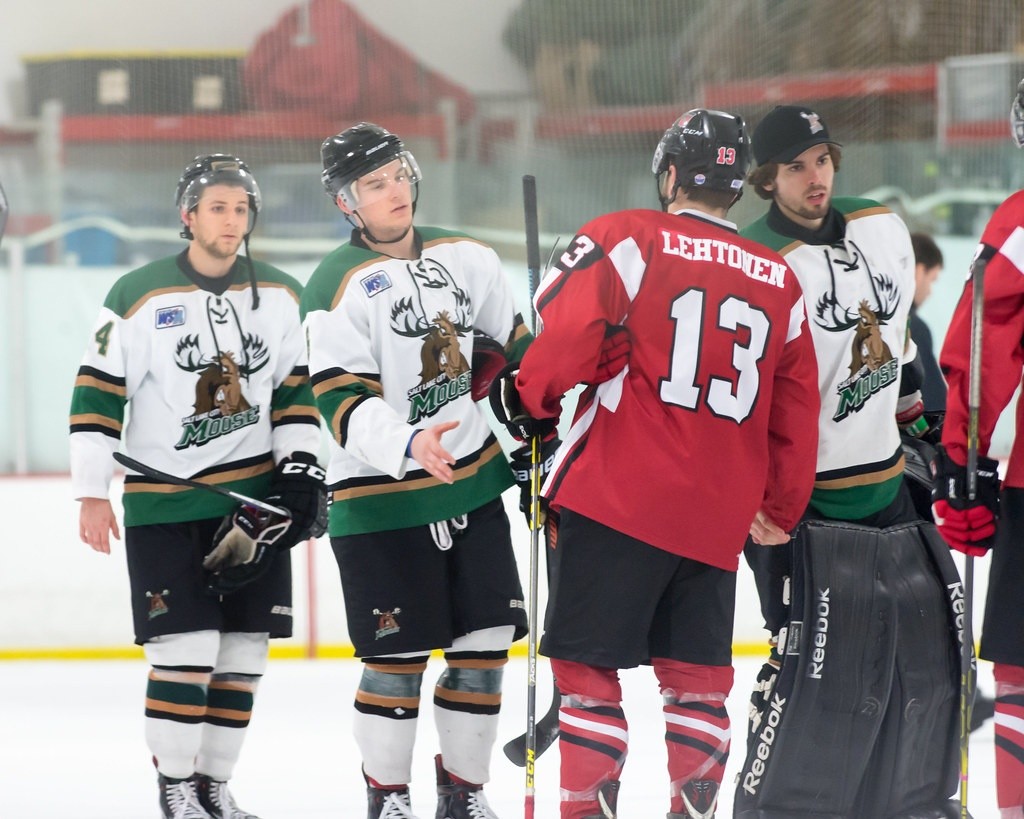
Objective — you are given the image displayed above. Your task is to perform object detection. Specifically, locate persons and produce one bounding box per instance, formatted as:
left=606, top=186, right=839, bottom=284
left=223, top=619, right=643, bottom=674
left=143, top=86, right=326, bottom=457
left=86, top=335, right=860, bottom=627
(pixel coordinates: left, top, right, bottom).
left=486, top=106, right=823, bottom=819
left=297, top=121, right=564, bottom=819
left=735, top=104, right=950, bottom=819
left=926, top=75, right=1024, bottom=819
left=66, top=150, right=334, bottom=819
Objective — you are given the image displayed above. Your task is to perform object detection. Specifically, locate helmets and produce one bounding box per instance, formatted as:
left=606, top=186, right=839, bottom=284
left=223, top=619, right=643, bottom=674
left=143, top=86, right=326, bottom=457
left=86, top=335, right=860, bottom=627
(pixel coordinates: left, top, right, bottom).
left=652, top=108, right=751, bottom=194
left=175, top=154, right=263, bottom=214
left=320, top=121, right=423, bottom=211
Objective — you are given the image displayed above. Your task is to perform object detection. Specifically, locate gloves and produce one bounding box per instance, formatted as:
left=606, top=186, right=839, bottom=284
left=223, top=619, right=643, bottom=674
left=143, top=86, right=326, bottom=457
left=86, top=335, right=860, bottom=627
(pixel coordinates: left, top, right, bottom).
left=929, top=443, right=1001, bottom=557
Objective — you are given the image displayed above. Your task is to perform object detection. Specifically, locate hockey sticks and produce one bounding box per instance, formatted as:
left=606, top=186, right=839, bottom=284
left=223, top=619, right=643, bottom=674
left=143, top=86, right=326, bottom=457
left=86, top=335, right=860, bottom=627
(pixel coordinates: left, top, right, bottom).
left=113, top=449, right=290, bottom=520
left=951, top=189, right=1023, bottom=818
left=504, top=233, right=563, bottom=769
left=517, top=174, right=558, bottom=818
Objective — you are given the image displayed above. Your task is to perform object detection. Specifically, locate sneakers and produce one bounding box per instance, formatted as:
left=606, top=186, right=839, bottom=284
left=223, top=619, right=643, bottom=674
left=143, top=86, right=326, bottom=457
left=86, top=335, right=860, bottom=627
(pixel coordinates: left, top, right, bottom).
left=194, top=772, right=261, bottom=819
left=152, top=756, right=214, bottom=819
left=362, top=762, right=418, bottom=819
left=435, top=754, right=499, bottom=819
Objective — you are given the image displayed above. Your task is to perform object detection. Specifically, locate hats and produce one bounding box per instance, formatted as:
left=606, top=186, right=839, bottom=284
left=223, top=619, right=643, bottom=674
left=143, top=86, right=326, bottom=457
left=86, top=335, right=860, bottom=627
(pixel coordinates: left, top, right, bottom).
left=751, top=105, right=843, bottom=167
